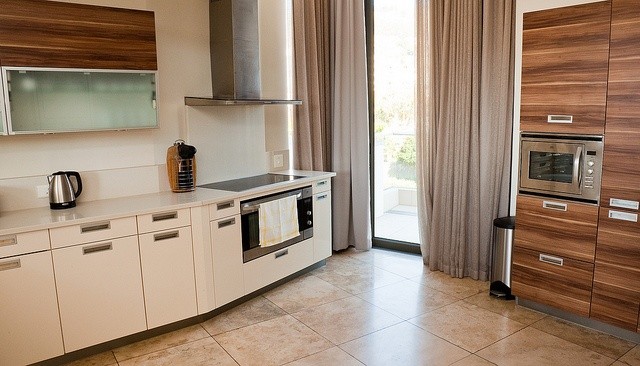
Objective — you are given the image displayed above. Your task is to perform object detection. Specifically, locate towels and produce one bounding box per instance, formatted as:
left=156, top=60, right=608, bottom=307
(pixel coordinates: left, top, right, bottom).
left=259, top=195, right=300, bottom=248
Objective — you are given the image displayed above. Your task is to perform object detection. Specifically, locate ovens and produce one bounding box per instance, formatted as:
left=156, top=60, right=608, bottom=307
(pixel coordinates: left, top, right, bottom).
left=239, top=184, right=321, bottom=263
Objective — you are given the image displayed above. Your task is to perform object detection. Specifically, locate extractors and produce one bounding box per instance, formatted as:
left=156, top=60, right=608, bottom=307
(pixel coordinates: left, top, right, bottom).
left=182, top=0, right=305, bottom=107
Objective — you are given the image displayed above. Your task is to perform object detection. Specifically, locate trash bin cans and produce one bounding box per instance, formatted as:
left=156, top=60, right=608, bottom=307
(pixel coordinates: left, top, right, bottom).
left=490, top=216, right=515, bottom=300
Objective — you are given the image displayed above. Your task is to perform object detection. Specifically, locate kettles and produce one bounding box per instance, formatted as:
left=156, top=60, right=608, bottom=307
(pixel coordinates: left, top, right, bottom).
left=46, top=171, right=84, bottom=210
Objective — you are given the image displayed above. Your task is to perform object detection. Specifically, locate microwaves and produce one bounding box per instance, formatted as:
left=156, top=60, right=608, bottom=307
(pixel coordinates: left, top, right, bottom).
left=519, top=132, right=605, bottom=206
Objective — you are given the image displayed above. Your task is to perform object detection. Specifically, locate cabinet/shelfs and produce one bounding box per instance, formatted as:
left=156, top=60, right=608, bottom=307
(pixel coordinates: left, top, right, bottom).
left=599, top=1, right=640, bottom=211
left=519, top=0, right=611, bottom=136
left=136, top=206, right=198, bottom=329
left=0, top=228, right=66, bottom=366
left=49, top=214, right=149, bottom=354
left=0, top=0, right=160, bottom=134
left=510, top=195, right=600, bottom=320
left=589, top=204, right=640, bottom=332
